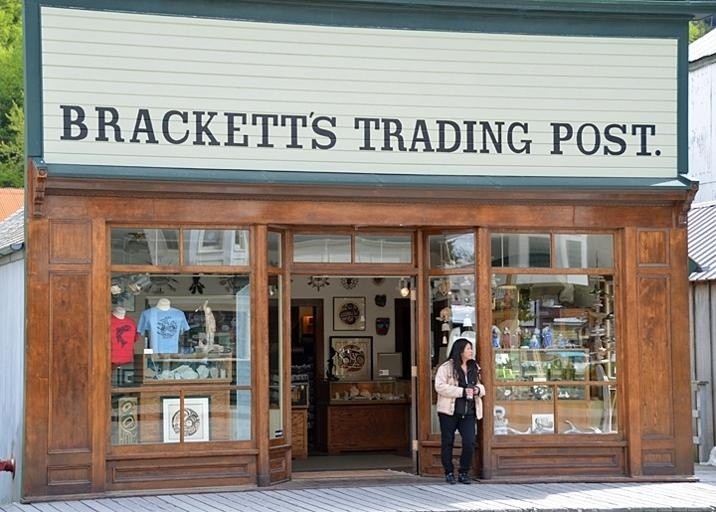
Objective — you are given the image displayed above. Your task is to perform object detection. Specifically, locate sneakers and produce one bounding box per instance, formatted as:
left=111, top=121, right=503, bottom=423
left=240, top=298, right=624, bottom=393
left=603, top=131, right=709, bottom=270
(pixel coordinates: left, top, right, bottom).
left=446, top=473, right=456, bottom=484
left=458, top=473, right=472, bottom=484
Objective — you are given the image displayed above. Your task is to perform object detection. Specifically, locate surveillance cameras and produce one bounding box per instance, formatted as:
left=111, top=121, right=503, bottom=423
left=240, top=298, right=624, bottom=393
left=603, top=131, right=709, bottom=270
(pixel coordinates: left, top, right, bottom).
left=678, top=174, right=699, bottom=190
left=32, top=158, right=48, bottom=178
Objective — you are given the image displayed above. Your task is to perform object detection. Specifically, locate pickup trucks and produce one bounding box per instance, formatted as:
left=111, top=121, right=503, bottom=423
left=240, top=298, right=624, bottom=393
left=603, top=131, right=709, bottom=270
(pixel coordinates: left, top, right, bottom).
left=520, top=351, right=590, bottom=381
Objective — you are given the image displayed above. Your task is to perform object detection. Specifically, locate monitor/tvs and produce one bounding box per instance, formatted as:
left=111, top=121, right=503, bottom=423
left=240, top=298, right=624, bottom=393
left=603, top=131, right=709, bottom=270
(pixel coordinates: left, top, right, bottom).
left=377, top=352, right=402, bottom=381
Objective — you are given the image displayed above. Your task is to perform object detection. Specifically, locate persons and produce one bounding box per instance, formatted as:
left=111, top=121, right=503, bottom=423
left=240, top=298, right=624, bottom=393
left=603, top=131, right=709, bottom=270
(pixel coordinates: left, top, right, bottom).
left=109, top=307, right=139, bottom=365
left=434, top=338, right=486, bottom=485
left=137, top=298, right=192, bottom=353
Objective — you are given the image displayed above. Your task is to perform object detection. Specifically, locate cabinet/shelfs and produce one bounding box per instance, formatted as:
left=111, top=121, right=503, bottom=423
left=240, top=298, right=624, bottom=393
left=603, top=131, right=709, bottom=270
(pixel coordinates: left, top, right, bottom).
left=493, top=346, right=592, bottom=428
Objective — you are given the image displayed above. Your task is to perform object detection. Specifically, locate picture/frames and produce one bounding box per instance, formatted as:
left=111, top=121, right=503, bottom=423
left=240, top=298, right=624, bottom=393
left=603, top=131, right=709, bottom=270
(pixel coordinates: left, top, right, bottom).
left=327, top=294, right=374, bottom=382
left=529, top=410, right=555, bottom=435
left=160, top=395, right=212, bottom=444
